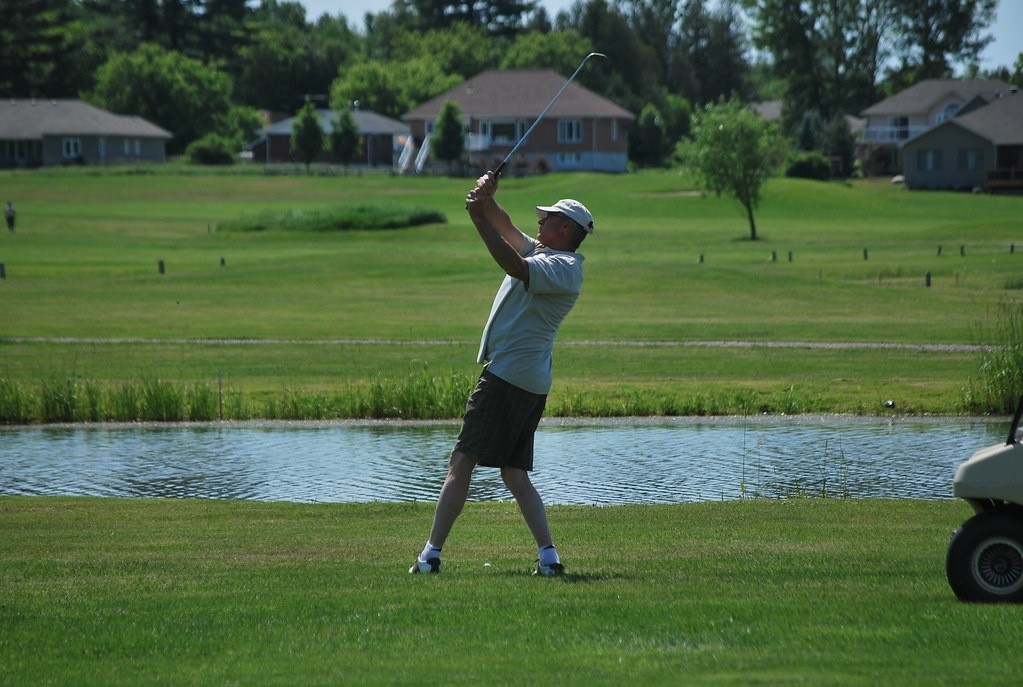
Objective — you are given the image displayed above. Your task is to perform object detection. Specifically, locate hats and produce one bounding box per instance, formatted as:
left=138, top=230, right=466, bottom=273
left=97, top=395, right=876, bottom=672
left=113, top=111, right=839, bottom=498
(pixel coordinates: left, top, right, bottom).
left=536, top=198, right=594, bottom=235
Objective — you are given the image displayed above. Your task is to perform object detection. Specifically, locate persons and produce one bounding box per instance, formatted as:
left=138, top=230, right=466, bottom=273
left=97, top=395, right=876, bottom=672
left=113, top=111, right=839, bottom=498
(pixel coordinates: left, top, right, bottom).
left=5, top=202, right=16, bottom=232
left=408, top=170, right=595, bottom=575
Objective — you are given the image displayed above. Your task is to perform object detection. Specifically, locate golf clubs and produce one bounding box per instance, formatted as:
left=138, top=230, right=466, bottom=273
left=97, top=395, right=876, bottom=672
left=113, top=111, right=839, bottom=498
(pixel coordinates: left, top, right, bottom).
left=491, top=52, right=609, bottom=177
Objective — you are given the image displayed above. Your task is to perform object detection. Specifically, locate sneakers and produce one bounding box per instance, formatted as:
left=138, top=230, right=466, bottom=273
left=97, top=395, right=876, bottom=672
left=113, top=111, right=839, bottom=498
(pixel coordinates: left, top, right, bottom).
left=408, top=554, right=441, bottom=575
left=531, top=558, right=566, bottom=577
